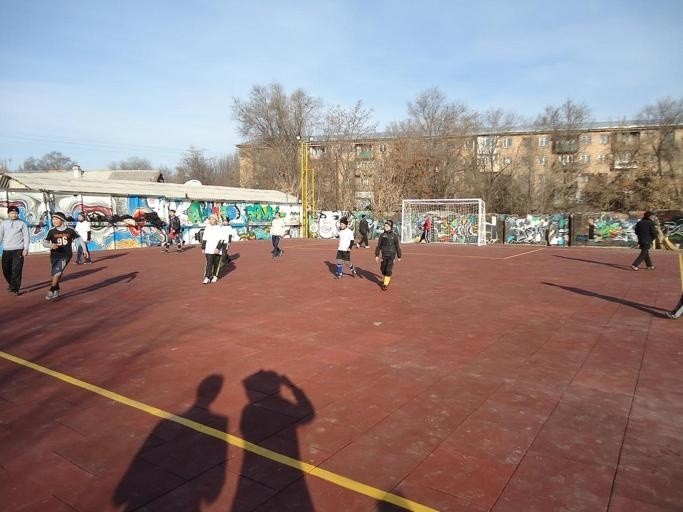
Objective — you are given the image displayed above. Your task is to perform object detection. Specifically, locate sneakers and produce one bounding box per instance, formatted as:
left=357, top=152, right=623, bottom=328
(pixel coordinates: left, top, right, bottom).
left=72, top=257, right=92, bottom=266
left=356, top=242, right=370, bottom=249
left=200, top=275, right=220, bottom=284
left=630, top=263, right=656, bottom=271
left=337, top=271, right=391, bottom=291
left=664, top=311, right=681, bottom=319
left=7, top=286, right=60, bottom=300
left=161, top=247, right=183, bottom=253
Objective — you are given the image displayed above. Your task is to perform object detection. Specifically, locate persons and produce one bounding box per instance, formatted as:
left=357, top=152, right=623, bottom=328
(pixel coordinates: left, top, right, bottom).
left=270, top=212, right=285, bottom=257
left=201, top=215, right=232, bottom=284
left=631, top=212, right=655, bottom=270
left=375, top=220, right=401, bottom=290
left=43, top=211, right=89, bottom=299
left=357, top=214, right=370, bottom=249
left=336, top=217, right=357, bottom=279
left=72, top=212, right=92, bottom=264
left=0, top=206, right=30, bottom=295
left=424, top=215, right=431, bottom=243
left=161, top=209, right=182, bottom=251
left=664, top=292, right=683, bottom=319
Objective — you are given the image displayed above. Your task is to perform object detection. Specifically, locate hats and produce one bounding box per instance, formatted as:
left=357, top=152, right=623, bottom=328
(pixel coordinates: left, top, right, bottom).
left=78, top=212, right=85, bottom=217
left=385, top=219, right=394, bottom=229
left=51, top=211, right=66, bottom=222
left=207, top=212, right=231, bottom=223
left=339, top=218, right=349, bottom=225
left=7, top=206, right=20, bottom=214
